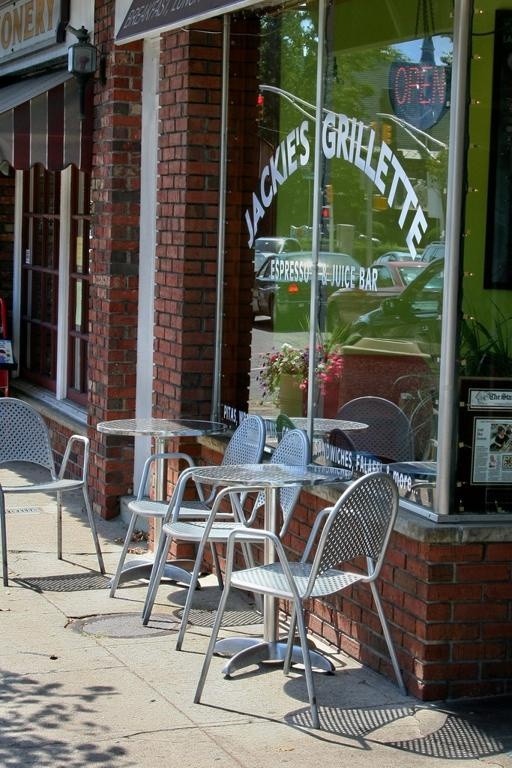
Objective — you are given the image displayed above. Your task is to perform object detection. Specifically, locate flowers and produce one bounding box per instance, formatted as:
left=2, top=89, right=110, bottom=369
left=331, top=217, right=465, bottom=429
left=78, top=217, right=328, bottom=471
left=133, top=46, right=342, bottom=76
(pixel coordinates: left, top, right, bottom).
left=257, top=313, right=360, bottom=405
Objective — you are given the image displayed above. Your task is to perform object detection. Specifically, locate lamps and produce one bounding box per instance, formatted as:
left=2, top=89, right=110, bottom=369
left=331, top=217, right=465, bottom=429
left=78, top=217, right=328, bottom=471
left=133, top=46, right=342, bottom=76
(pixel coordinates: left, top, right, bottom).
left=65, top=24, right=107, bottom=121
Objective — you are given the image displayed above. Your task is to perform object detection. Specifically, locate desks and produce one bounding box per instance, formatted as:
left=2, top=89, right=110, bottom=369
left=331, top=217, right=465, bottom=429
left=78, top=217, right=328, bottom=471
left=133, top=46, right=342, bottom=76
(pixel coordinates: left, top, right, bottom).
left=188, top=461, right=354, bottom=678
left=363, top=460, right=437, bottom=480
left=98, top=419, right=230, bottom=594
left=263, top=414, right=369, bottom=433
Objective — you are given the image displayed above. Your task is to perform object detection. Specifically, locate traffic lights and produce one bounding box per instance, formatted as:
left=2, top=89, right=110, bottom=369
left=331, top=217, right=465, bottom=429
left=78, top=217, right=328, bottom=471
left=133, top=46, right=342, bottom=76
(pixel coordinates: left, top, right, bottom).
left=383, top=124, right=392, bottom=143
left=369, top=122, right=377, bottom=141
left=323, top=185, right=331, bottom=203
left=322, top=208, right=330, bottom=221
left=256, top=96, right=264, bottom=120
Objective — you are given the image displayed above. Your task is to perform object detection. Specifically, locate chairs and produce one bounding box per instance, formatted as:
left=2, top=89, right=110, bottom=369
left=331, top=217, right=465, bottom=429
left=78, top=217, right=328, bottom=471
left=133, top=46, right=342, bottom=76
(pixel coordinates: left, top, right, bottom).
left=143, top=430, right=309, bottom=655
left=275, top=411, right=294, bottom=443
left=325, top=427, right=358, bottom=476
left=193, top=470, right=409, bottom=728
left=0, top=397, right=106, bottom=586
left=323, top=392, right=424, bottom=474
left=111, top=417, right=268, bottom=602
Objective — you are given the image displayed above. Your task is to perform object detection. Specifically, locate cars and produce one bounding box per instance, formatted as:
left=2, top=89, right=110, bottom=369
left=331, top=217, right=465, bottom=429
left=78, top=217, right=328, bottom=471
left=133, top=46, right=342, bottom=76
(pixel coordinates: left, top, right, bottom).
left=252, top=232, right=445, bottom=334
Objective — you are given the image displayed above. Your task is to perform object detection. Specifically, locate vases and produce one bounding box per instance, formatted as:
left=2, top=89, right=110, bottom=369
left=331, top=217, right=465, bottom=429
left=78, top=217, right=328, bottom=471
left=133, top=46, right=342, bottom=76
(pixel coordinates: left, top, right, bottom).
left=275, top=371, right=306, bottom=417
